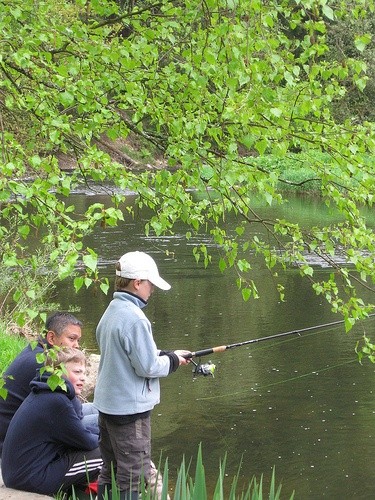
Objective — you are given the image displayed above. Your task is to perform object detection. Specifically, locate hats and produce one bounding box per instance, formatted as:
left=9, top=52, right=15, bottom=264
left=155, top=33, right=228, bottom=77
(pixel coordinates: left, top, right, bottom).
left=116, top=251, right=172, bottom=290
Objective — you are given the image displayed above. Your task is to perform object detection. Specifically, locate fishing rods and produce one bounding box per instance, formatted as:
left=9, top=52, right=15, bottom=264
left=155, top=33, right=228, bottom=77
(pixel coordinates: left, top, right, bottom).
left=181, top=312, right=375, bottom=376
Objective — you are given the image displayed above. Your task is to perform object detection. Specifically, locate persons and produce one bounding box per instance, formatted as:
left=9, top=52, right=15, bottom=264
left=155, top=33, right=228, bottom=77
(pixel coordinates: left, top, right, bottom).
left=0, top=347, right=103, bottom=500
left=93, top=250, right=193, bottom=500
left=0, top=312, right=101, bottom=459
